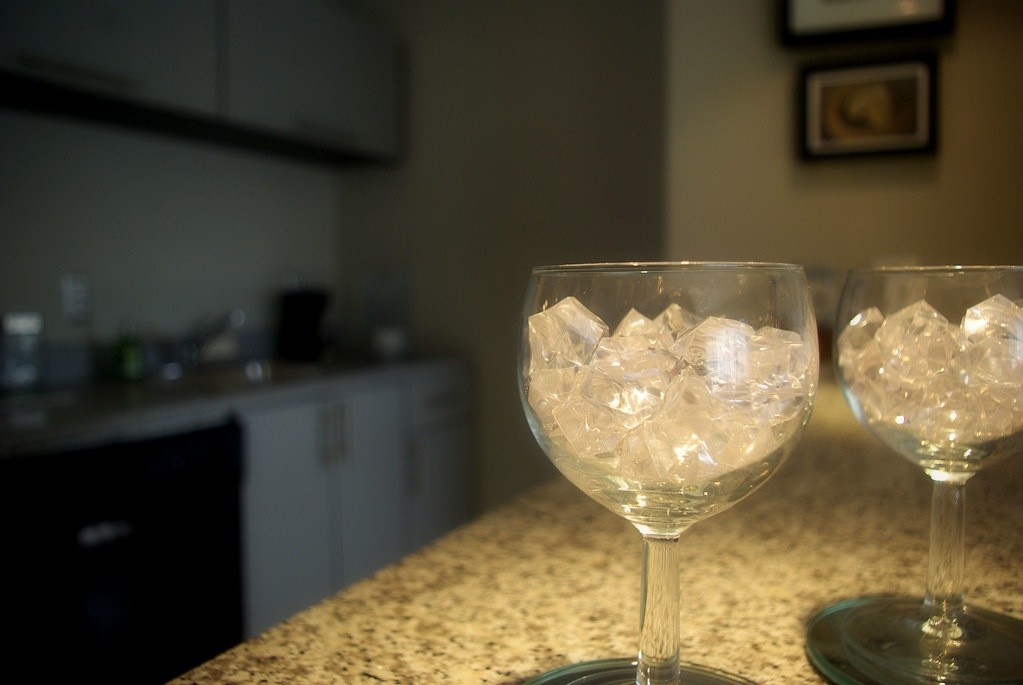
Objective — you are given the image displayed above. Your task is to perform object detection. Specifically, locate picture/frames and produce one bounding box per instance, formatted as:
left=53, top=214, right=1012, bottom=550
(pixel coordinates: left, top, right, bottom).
left=776, top=0, right=956, bottom=49
left=796, top=46, right=938, bottom=159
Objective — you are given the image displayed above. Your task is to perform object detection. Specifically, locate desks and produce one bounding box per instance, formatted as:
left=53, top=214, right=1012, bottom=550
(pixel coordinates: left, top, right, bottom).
left=169, top=358, right=1022, bottom=685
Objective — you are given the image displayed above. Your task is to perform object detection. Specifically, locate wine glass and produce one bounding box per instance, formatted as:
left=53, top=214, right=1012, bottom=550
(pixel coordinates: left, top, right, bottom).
left=804, top=266, right=1023, bottom=685
left=510, top=260, right=822, bottom=685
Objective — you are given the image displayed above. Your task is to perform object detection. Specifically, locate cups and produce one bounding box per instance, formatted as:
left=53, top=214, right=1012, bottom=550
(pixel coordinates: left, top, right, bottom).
left=2, top=311, right=45, bottom=390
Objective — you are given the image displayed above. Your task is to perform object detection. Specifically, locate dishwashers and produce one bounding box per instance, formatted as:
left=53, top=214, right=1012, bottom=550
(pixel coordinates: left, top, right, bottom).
left=0, top=411, right=255, bottom=685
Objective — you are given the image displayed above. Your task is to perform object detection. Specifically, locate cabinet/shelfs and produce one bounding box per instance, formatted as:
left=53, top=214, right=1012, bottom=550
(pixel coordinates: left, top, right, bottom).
left=0, top=0, right=412, bottom=164
left=239, top=372, right=479, bottom=642
